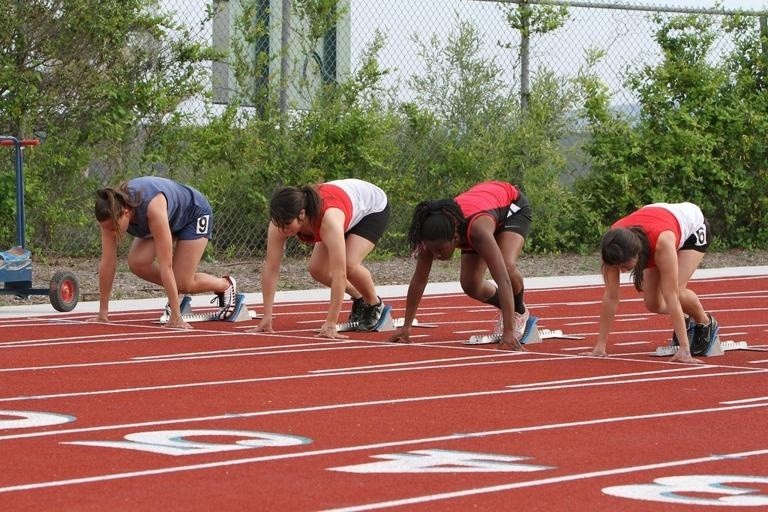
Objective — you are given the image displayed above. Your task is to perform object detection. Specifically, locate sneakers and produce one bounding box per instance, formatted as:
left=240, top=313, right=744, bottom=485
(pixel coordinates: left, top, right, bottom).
left=159, top=289, right=186, bottom=324
left=362, top=294, right=385, bottom=329
left=215, top=271, right=237, bottom=321
left=670, top=310, right=720, bottom=356
left=347, top=294, right=364, bottom=324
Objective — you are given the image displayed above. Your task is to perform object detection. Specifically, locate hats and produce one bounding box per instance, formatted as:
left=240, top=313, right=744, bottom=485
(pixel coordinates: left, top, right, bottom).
left=491, top=302, right=531, bottom=342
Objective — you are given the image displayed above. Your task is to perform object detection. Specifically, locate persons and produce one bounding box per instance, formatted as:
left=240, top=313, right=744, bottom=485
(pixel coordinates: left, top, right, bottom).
left=385, top=180, right=534, bottom=352
left=244, top=178, right=392, bottom=340
left=576, top=201, right=721, bottom=365
left=86, top=175, right=238, bottom=330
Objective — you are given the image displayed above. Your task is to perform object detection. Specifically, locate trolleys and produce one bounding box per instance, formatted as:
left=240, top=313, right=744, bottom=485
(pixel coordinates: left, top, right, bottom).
left=0, top=129, right=82, bottom=313
